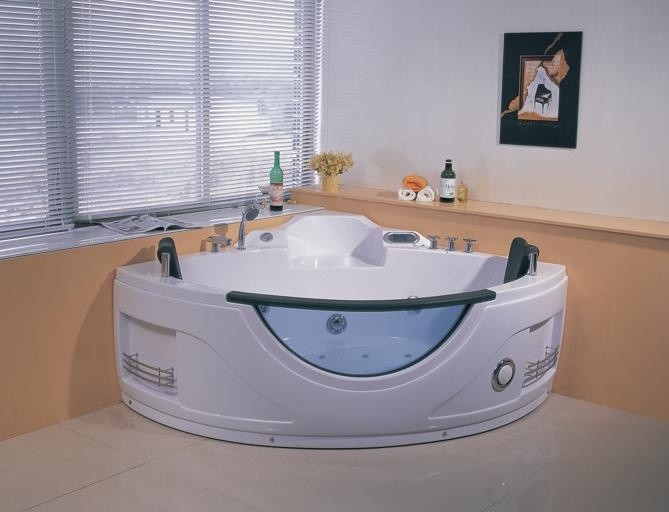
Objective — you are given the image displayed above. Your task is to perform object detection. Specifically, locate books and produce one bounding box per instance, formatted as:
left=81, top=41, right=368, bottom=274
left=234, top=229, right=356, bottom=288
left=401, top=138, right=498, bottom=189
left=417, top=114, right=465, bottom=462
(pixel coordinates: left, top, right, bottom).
left=99, top=215, right=203, bottom=235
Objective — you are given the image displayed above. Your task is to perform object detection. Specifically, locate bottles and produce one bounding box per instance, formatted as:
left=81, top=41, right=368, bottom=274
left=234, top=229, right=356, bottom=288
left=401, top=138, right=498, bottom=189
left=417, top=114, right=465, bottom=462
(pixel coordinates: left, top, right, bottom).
left=439, top=160, right=456, bottom=202
left=270, top=152, right=285, bottom=211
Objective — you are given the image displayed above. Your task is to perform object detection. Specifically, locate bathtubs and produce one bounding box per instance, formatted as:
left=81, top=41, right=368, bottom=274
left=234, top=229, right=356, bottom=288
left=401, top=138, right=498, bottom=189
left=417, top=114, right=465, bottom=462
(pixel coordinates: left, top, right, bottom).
left=113, top=209, right=567, bottom=449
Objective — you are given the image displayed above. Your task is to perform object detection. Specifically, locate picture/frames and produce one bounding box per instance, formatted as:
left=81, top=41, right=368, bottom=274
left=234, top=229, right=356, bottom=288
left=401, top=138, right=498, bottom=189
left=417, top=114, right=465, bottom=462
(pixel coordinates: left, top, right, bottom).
left=500, top=31, right=583, bottom=148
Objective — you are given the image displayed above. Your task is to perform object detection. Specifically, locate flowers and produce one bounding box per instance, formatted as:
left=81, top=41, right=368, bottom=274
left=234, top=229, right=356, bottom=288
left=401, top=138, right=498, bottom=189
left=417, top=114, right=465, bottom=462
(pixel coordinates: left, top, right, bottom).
left=309, top=149, right=353, bottom=176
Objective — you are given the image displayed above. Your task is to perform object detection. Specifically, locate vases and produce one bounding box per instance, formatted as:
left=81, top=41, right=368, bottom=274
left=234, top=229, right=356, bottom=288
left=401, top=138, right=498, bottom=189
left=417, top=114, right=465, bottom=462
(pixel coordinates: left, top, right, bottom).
left=322, top=174, right=338, bottom=192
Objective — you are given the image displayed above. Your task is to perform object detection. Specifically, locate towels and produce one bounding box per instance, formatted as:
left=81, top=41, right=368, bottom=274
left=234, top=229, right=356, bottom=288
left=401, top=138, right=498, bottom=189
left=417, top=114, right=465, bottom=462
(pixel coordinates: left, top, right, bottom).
left=398, top=185, right=416, bottom=201
left=416, top=186, right=435, bottom=201
left=402, top=174, right=427, bottom=193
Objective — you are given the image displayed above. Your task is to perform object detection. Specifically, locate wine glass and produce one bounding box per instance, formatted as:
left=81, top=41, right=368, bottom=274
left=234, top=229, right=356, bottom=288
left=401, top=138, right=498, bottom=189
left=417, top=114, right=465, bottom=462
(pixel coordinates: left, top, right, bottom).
left=257, top=186, right=271, bottom=205
